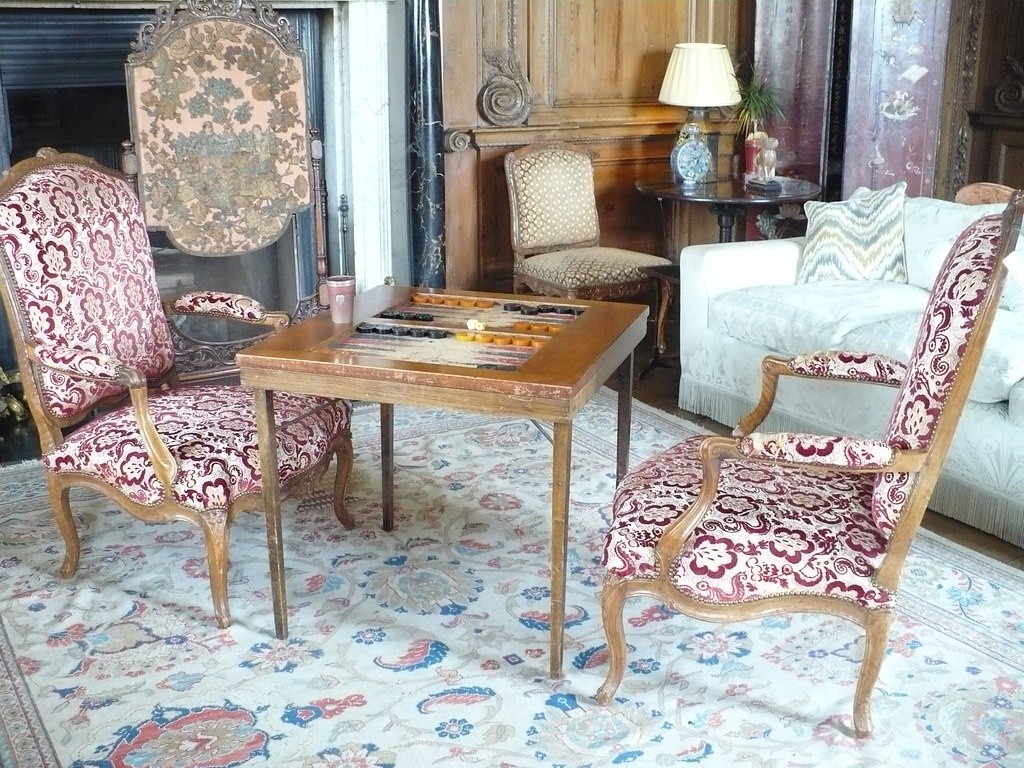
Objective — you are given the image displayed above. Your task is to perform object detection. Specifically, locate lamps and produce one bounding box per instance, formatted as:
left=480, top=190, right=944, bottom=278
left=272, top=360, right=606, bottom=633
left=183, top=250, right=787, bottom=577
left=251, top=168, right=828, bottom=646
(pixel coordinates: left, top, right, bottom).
left=657, top=42, right=742, bottom=184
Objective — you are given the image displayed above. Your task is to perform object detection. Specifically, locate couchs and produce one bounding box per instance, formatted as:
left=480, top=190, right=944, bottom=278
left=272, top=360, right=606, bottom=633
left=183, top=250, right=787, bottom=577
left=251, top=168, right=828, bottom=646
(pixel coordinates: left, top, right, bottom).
left=679, top=182, right=1023, bottom=550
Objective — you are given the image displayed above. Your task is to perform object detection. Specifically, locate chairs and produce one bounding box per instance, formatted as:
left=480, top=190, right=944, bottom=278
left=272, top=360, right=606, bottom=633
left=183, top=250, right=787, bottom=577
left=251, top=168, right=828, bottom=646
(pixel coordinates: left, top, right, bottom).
left=0, top=145, right=356, bottom=628
left=594, top=191, right=1024, bottom=740
left=502, top=139, right=672, bottom=353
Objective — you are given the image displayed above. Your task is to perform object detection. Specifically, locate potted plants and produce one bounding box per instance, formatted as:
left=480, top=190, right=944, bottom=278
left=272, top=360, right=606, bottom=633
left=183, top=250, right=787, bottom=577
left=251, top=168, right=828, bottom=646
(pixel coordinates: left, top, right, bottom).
left=723, top=60, right=786, bottom=175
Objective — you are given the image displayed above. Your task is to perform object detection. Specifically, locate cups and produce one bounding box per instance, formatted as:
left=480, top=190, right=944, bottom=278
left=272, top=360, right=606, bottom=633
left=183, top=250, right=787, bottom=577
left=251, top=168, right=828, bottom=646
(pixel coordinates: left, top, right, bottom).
left=327, top=276, right=355, bottom=324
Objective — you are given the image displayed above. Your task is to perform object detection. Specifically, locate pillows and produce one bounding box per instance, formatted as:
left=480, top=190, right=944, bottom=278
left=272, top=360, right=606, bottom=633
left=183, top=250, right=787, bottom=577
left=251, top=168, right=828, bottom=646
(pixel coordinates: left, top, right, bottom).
left=911, top=219, right=1023, bottom=315
left=799, top=180, right=909, bottom=283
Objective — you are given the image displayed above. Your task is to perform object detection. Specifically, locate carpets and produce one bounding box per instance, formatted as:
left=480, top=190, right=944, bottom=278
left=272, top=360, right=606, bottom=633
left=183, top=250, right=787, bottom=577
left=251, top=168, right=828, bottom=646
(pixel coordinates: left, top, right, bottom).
left=0, top=389, right=1023, bottom=768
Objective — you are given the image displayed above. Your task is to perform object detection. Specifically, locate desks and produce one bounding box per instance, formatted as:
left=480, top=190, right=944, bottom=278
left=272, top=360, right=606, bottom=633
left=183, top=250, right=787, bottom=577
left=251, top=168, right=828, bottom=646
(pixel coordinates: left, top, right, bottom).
left=630, top=174, right=822, bottom=354
left=233, top=284, right=648, bottom=680
